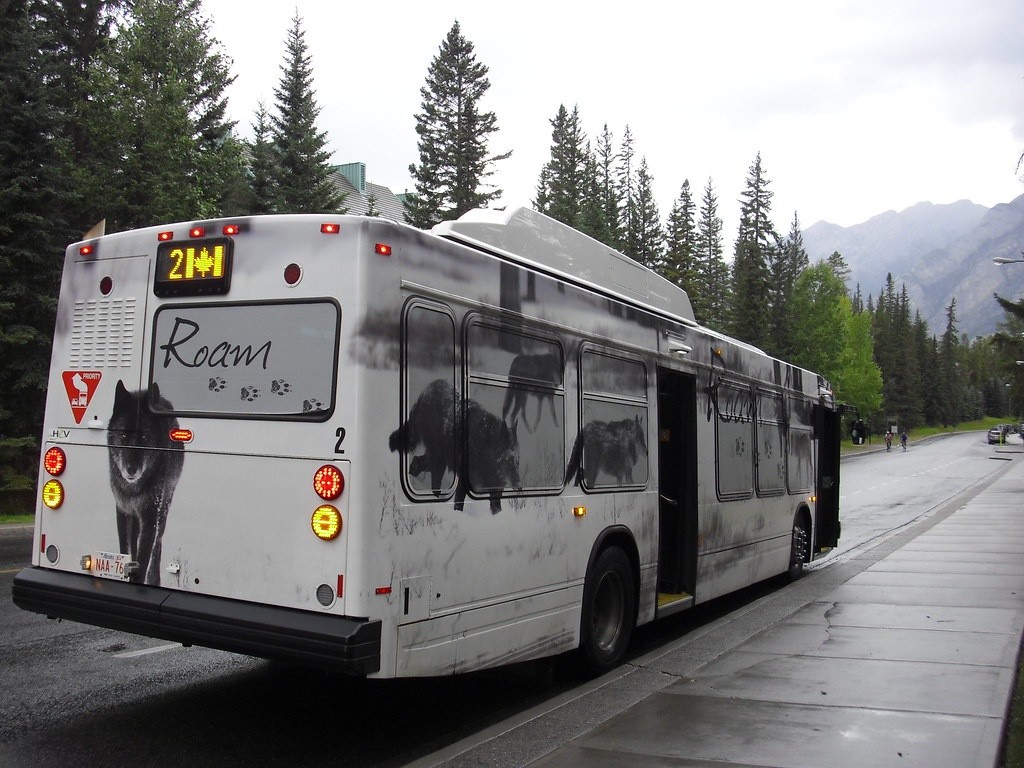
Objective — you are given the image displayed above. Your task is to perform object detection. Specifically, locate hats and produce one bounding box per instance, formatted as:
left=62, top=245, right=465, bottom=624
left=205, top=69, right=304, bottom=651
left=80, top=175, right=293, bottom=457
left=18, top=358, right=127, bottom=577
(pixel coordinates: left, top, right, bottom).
left=902, top=432, right=905, bottom=435
left=886, top=431, right=890, bottom=433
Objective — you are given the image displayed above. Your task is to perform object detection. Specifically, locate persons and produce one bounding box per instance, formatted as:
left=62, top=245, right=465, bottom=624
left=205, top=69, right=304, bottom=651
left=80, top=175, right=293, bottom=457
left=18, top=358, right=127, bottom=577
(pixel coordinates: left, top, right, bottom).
left=900, top=432, right=907, bottom=448
left=884, top=431, right=892, bottom=449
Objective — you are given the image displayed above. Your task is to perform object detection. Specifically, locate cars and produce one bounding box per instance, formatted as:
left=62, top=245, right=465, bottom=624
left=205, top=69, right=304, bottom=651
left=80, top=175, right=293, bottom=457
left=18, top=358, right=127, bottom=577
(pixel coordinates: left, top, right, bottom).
left=987, top=422, right=1024, bottom=443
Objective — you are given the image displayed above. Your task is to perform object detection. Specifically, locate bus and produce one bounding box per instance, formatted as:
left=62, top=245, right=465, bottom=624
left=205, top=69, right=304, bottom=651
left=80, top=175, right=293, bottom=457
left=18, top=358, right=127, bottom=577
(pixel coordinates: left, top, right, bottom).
left=10, top=200, right=866, bottom=682
